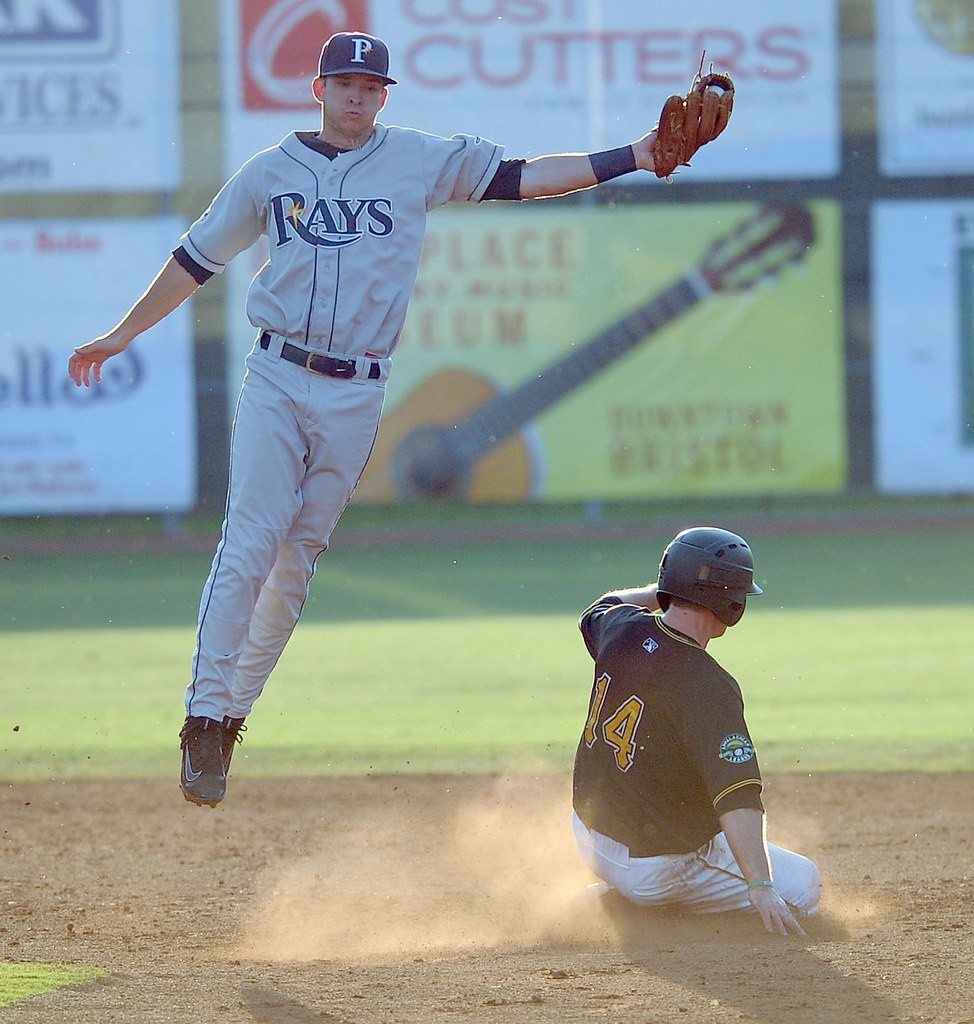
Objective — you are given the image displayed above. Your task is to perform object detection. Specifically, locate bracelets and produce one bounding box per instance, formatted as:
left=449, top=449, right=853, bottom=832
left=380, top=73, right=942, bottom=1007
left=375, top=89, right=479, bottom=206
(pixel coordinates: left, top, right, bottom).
left=747, top=880, right=773, bottom=888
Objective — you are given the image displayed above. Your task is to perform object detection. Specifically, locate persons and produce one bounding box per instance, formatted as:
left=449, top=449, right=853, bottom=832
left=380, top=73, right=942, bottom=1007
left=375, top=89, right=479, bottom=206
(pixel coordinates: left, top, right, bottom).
left=69, top=36, right=736, bottom=807
left=573, top=527, right=822, bottom=937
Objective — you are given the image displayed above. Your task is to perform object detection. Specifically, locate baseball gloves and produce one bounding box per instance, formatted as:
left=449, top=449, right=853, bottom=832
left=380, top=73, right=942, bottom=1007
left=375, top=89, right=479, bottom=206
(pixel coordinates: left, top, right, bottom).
left=653, top=72, right=736, bottom=179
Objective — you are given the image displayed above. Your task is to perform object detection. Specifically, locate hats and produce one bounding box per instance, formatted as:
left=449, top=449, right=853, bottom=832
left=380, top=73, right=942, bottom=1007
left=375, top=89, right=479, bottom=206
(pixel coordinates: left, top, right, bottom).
left=317, top=30, right=398, bottom=85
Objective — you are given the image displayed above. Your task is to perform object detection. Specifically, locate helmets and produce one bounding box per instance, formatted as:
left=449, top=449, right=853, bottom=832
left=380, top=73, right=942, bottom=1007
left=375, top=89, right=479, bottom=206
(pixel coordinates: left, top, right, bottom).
left=653, top=525, right=764, bottom=628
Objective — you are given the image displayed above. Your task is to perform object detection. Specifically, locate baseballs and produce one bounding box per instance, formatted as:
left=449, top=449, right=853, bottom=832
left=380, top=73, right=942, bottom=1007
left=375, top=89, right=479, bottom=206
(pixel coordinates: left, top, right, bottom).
left=703, top=86, right=725, bottom=99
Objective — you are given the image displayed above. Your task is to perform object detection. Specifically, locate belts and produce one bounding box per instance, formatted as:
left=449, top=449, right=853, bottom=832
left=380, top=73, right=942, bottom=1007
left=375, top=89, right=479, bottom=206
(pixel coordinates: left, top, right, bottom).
left=260, top=331, right=380, bottom=379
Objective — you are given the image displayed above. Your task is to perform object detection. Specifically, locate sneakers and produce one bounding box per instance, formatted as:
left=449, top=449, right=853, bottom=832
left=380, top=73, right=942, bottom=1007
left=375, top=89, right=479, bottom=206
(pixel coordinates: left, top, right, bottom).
left=178, top=714, right=248, bottom=808
